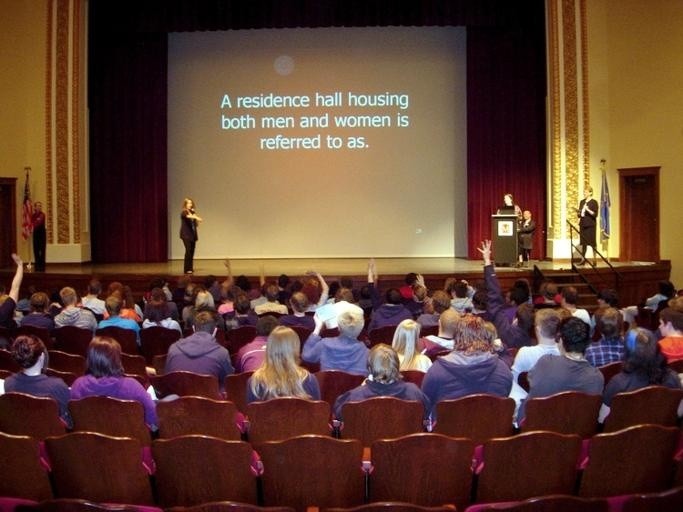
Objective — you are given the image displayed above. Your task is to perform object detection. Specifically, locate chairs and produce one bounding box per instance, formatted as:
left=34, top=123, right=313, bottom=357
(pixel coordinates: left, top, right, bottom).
left=419, top=324, right=440, bottom=335
left=2, top=348, right=17, bottom=373
left=96, top=326, right=141, bottom=353
left=367, top=325, right=396, bottom=344
left=520, top=392, right=604, bottom=428
left=155, top=397, right=241, bottom=440
left=2, top=433, right=51, bottom=501
left=1, top=369, right=14, bottom=380
left=40, top=498, right=99, bottom=507
left=506, top=495, right=608, bottom=507
left=39, top=368, right=78, bottom=389
left=68, top=395, right=151, bottom=445
left=155, top=435, right=254, bottom=502
left=0, top=392, right=64, bottom=433
left=156, top=371, right=220, bottom=397
left=580, top=423, right=681, bottom=498
left=340, top=395, right=426, bottom=438
left=291, top=324, right=313, bottom=346
left=479, top=430, right=583, bottom=497
left=315, top=369, right=365, bottom=406
left=246, top=397, right=333, bottom=445
left=16, top=325, right=54, bottom=348
left=431, top=392, right=516, bottom=438
left=55, top=326, right=95, bottom=357
left=400, top=371, right=425, bottom=387
left=44, top=432, right=152, bottom=502
left=228, top=325, right=258, bottom=353
left=623, top=487, right=682, bottom=508
left=47, top=349, right=84, bottom=372
left=124, top=371, right=150, bottom=383
left=141, top=326, right=182, bottom=364
left=371, top=433, right=476, bottom=503
left=122, top=352, right=148, bottom=373
left=596, top=361, right=624, bottom=377
left=223, top=371, right=255, bottom=409
left=604, top=385, right=683, bottom=431
left=258, top=436, right=368, bottom=507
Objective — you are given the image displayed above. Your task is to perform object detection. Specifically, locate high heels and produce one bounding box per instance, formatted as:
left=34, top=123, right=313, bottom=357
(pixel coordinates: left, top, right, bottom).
left=576, top=260, right=585, bottom=266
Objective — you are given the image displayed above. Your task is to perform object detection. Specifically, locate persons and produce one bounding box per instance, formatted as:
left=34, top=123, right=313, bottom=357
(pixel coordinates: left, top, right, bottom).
left=22, top=201, right=46, bottom=272
left=561, top=286, right=596, bottom=338
left=577, top=185, right=598, bottom=266
left=422, top=316, right=513, bottom=419
left=496, top=193, right=523, bottom=238
left=512, top=279, right=532, bottom=304
left=644, top=282, right=683, bottom=364
left=246, top=327, right=322, bottom=404
left=318, top=257, right=477, bottom=327
left=597, top=327, right=682, bottom=432
left=533, top=282, right=561, bottom=311
left=485, top=288, right=523, bottom=321
left=236, top=316, right=279, bottom=375
left=0, top=253, right=194, bottom=337
left=421, top=309, right=460, bottom=365
left=477, top=240, right=536, bottom=347
left=70, top=337, right=159, bottom=432
left=508, top=308, right=561, bottom=418
left=586, top=307, right=627, bottom=367
left=163, top=311, right=234, bottom=394
left=516, top=210, right=535, bottom=268
left=192, top=258, right=317, bottom=327
left=590, top=288, right=625, bottom=338
left=517, top=316, right=606, bottom=428
left=334, top=343, right=430, bottom=423
left=0, top=335, right=73, bottom=430
left=179, top=198, right=202, bottom=275
left=391, top=320, right=433, bottom=374
left=303, top=311, right=371, bottom=378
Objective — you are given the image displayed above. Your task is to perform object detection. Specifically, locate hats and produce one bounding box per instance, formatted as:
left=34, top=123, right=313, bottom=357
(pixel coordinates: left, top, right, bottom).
left=540, top=282, right=557, bottom=299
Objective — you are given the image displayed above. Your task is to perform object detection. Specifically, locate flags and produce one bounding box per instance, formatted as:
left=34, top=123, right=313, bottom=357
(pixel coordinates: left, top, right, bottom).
left=599, top=170, right=611, bottom=251
left=21, top=174, right=33, bottom=241
